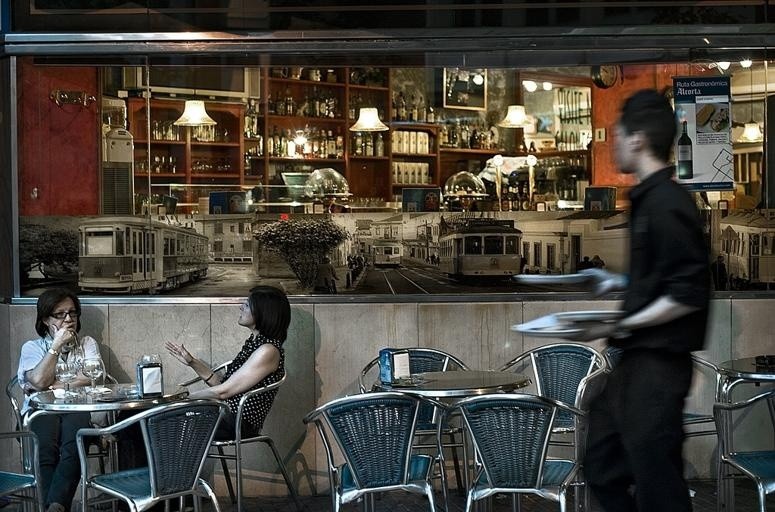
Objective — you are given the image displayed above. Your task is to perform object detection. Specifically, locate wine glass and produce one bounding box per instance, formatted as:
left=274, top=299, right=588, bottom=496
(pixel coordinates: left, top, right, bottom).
left=82, top=358, right=103, bottom=396
left=55, top=362, right=80, bottom=401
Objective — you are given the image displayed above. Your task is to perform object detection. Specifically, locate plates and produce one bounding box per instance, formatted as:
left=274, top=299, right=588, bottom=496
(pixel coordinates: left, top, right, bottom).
left=516, top=274, right=594, bottom=286
left=558, top=310, right=624, bottom=321
left=509, top=323, right=585, bottom=339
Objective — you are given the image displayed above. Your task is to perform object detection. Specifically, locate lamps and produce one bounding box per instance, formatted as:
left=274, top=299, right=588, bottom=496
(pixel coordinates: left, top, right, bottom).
left=497, top=106, right=532, bottom=128
left=743, top=67, right=761, bottom=139
left=349, top=84, right=389, bottom=131
left=174, top=90, right=216, bottom=125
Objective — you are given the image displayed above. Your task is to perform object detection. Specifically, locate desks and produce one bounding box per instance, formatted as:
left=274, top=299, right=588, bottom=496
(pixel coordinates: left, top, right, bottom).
left=372, top=368, right=537, bottom=512
left=717, top=356, right=775, bottom=512
left=27, top=384, right=190, bottom=510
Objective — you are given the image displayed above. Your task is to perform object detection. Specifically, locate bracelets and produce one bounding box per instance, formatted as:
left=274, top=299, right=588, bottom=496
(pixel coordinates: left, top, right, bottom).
left=49, top=349, right=60, bottom=357
left=201, top=373, right=214, bottom=382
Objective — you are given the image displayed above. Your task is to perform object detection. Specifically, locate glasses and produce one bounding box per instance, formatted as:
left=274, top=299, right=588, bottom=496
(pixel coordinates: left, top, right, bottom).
left=48, top=309, right=80, bottom=320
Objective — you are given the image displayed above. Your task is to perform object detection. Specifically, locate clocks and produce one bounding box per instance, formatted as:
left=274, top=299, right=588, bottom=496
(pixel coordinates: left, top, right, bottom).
left=591, top=66, right=619, bottom=89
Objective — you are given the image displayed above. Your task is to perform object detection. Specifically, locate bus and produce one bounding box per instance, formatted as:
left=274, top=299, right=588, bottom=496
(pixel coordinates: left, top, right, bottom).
left=372, top=239, right=402, bottom=267
left=77, top=216, right=209, bottom=294
left=435, top=217, right=523, bottom=279
left=718, top=209, right=775, bottom=285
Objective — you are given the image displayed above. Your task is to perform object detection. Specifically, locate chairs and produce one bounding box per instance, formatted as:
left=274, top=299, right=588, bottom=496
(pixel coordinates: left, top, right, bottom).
left=596, top=347, right=724, bottom=512
left=2, top=431, right=43, bottom=512
left=177, top=357, right=286, bottom=511
left=6, top=372, right=117, bottom=510
left=448, top=394, right=593, bottom=511
left=713, top=391, right=775, bottom=512
left=356, top=347, right=470, bottom=499
left=492, top=343, right=605, bottom=512
left=304, top=390, right=446, bottom=512
left=77, top=399, right=231, bottom=511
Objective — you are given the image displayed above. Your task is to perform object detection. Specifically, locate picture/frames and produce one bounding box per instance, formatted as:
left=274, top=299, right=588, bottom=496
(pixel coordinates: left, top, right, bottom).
left=442, top=68, right=487, bottom=111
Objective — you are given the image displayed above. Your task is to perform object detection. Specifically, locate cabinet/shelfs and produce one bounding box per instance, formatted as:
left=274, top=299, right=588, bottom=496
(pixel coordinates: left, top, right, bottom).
left=244, top=66, right=439, bottom=200
left=128, top=97, right=243, bottom=213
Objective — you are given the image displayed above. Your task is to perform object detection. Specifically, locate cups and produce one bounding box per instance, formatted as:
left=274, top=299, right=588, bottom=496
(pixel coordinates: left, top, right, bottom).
left=152, top=120, right=220, bottom=142
left=141, top=353, right=163, bottom=365
left=347, top=197, right=386, bottom=206
left=391, top=131, right=431, bottom=184
left=137, top=152, right=232, bottom=173
left=273, top=65, right=338, bottom=81
left=385, top=202, right=402, bottom=209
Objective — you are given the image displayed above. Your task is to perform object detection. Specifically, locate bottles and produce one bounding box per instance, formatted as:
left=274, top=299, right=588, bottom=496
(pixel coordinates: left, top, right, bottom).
left=392, top=93, right=434, bottom=123
left=500, top=156, right=587, bottom=214
left=437, top=113, right=494, bottom=150
left=444, top=184, right=486, bottom=212
left=245, top=99, right=258, bottom=137
left=312, top=189, right=324, bottom=214
left=348, top=92, right=385, bottom=158
left=677, top=121, right=693, bottom=179
left=268, top=84, right=344, bottom=160
left=244, top=152, right=251, bottom=175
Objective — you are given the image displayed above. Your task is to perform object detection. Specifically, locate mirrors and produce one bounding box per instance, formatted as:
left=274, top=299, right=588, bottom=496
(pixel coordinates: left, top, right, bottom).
left=517, top=70, right=593, bottom=152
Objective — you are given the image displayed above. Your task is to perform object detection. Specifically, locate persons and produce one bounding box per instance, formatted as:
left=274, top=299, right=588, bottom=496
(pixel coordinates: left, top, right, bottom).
left=117, top=285, right=292, bottom=512
left=18, top=288, right=106, bottom=512
left=564, top=87, right=715, bottom=512
